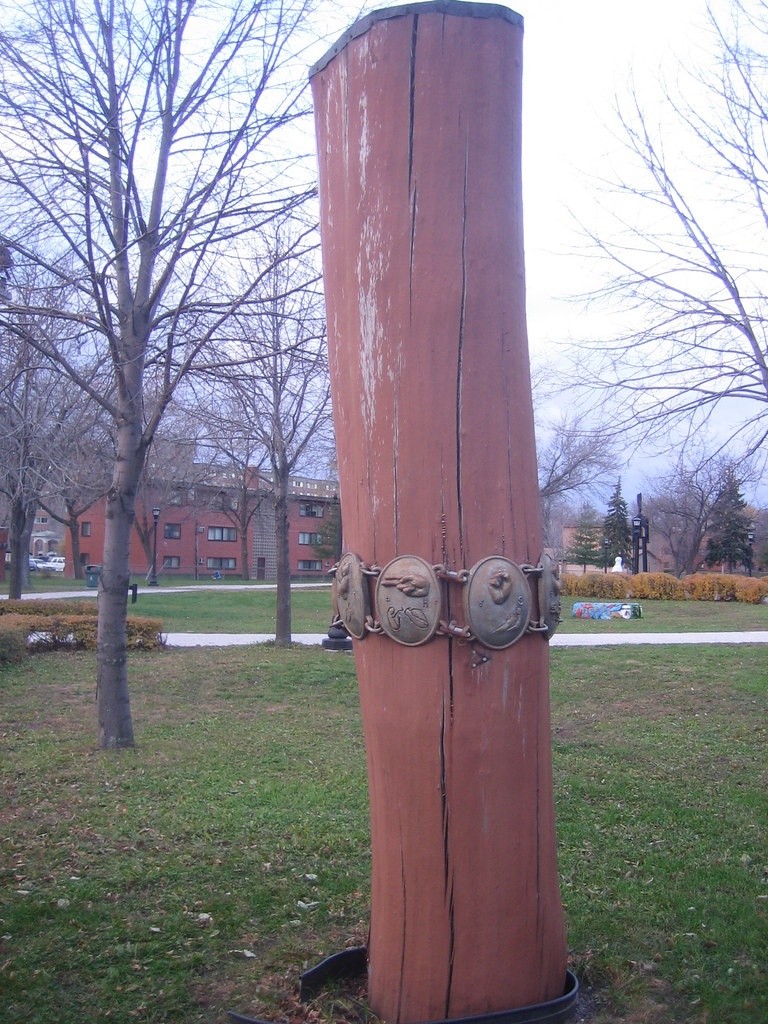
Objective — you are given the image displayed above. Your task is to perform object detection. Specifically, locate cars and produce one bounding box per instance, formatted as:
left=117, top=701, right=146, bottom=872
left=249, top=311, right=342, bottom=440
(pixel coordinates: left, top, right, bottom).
left=28, top=551, right=65, bottom=573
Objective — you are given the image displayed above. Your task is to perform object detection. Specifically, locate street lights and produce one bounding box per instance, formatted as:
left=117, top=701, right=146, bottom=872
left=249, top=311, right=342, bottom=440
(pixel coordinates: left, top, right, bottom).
left=747, top=532, right=755, bottom=577
left=632, top=516, right=641, bottom=577
left=147, top=507, right=163, bottom=587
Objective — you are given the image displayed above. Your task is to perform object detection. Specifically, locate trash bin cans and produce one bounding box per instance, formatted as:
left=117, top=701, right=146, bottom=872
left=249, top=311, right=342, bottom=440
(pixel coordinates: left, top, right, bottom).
left=84, top=565, right=103, bottom=589
